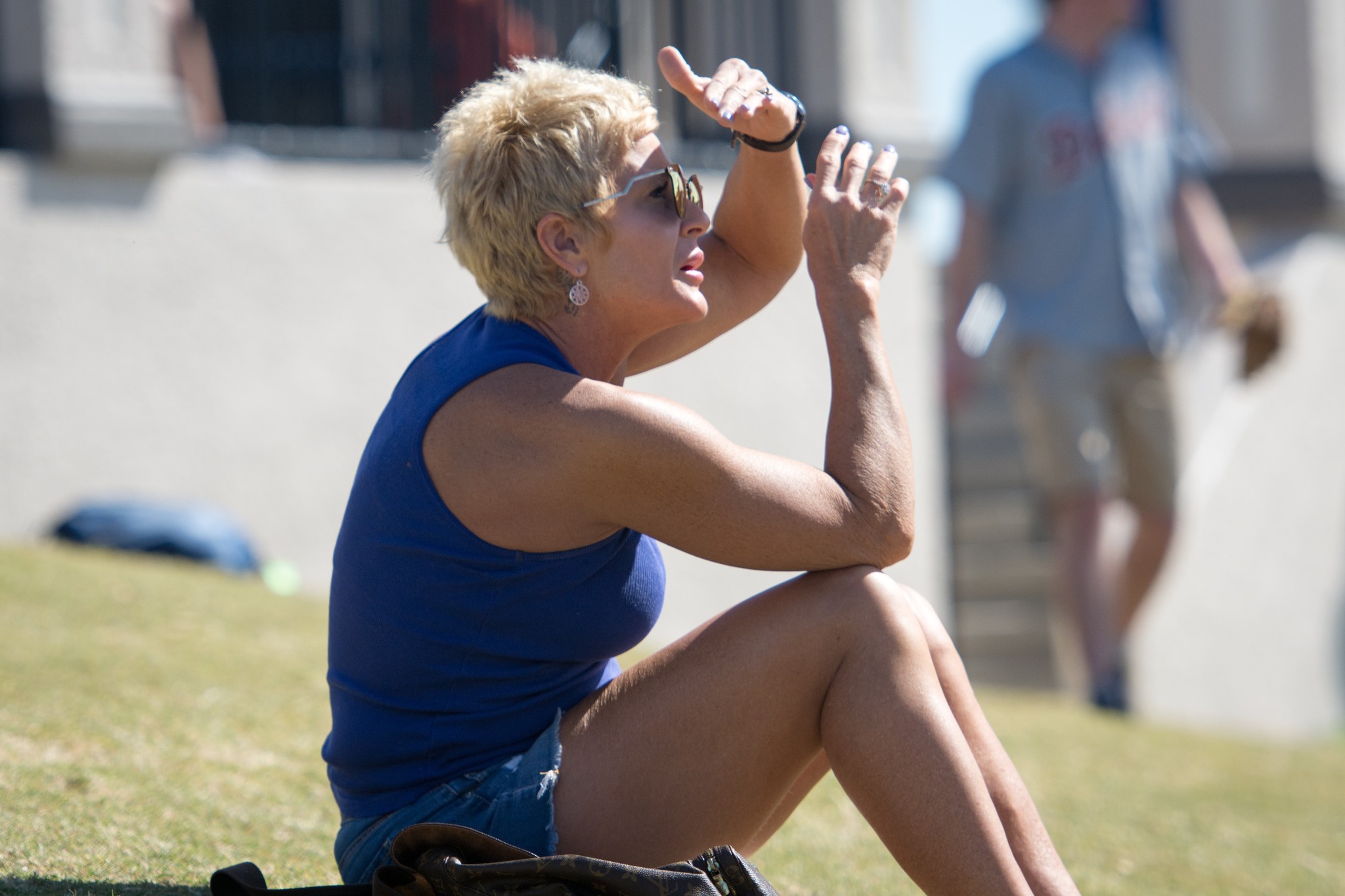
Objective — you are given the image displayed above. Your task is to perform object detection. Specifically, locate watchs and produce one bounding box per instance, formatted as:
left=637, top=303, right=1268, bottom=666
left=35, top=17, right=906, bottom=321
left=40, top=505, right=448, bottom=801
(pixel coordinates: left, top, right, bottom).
left=730, top=89, right=807, bottom=152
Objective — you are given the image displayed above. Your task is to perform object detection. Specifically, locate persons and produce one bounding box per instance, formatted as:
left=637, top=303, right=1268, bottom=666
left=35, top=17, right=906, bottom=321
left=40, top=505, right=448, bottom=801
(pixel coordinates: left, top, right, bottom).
left=322, top=43, right=1082, bottom=896
left=936, top=0, right=1261, bottom=713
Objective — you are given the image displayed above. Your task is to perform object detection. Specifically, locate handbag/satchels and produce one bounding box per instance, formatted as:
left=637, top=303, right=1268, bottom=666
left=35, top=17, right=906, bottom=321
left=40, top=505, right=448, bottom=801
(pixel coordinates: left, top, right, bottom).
left=372, top=822, right=779, bottom=896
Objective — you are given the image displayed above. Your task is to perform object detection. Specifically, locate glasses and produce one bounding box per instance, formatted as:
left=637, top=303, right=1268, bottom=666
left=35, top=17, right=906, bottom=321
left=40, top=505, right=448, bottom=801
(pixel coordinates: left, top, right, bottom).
left=571, top=165, right=703, bottom=217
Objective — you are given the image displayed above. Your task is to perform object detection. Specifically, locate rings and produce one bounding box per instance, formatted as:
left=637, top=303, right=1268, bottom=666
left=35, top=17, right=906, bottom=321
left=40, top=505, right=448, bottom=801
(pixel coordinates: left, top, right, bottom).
left=757, top=86, right=773, bottom=97
left=864, top=178, right=890, bottom=197
